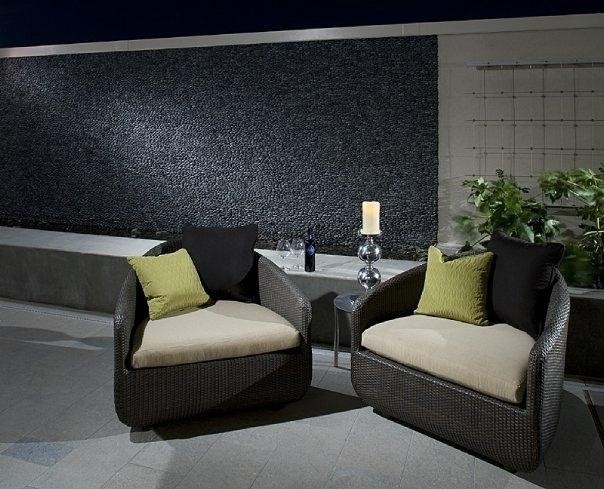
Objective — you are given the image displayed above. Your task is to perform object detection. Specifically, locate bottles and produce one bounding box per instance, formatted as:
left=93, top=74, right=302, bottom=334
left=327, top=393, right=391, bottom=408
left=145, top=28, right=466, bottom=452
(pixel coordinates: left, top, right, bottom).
left=305, top=227, right=316, bottom=273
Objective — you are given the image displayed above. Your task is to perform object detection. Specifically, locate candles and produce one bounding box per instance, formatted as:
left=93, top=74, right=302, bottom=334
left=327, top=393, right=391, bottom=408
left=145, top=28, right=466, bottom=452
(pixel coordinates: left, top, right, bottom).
left=361, top=200, right=382, bottom=235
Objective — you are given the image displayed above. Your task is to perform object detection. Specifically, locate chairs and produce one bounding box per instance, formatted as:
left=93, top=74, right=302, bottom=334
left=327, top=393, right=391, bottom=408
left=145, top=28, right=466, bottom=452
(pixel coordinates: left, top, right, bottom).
left=350, top=248, right=571, bottom=473
left=114, top=235, right=313, bottom=428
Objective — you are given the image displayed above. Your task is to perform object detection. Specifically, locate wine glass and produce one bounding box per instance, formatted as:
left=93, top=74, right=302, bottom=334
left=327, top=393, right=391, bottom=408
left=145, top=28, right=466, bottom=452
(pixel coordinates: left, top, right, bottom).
left=276, top=238, right=305, bottom=271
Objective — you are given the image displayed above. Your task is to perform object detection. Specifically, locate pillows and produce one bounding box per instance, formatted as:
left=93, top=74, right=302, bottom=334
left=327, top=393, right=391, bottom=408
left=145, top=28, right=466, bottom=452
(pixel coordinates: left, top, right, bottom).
left=126, top=248, right=216, bottom=321
left=485, top=229, right=565, bottom=342
left=182, top=221, right=259, bottom=303
left=414, top=245, right=495, bottom=326
